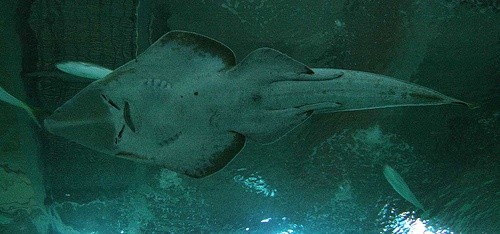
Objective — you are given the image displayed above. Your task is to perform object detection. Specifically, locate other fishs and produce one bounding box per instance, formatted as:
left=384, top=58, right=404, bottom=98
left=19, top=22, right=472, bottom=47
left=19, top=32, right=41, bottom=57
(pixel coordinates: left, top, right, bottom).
left=55, top=60, right=116, bottom=80
left=0, top=86, right=42, bottom=128
left=378, top=163, right=426, bottom=214
left=44, top=30, right=479, bottom=180
